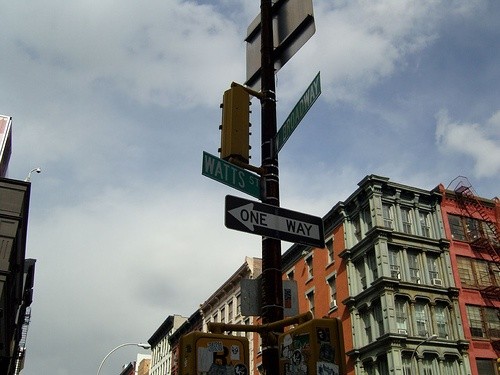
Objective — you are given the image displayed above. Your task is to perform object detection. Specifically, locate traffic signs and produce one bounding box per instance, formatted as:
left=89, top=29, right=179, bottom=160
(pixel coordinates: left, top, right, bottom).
left=245, top=1, right=317, bottom=98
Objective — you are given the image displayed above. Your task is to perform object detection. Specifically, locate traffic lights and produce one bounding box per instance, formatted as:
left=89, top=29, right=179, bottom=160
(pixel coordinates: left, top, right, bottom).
left=278, top=318, right=346, bottom=375
left=178, top=332, right=249, bottom=375
left=218, top=86, right=251, bottom=170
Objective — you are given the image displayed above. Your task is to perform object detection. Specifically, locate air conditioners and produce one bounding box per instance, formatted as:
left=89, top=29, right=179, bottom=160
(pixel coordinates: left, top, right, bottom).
left=431, top=278, right=442, bottom=286
left=329, top=300, right=337, bottom=309
left=398, top=330, right=406, bottom=334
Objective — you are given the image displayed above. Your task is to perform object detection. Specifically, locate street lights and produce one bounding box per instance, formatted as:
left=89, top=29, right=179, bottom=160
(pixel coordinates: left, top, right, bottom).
left=97, top=342, right=151, bottom=374
